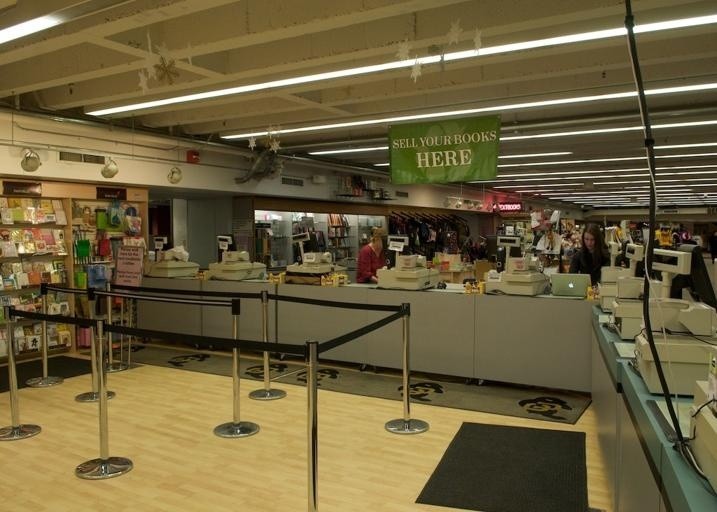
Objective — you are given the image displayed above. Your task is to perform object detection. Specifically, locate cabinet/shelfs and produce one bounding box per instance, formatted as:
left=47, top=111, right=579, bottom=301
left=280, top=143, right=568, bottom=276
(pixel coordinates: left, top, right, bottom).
left=0, top=194, right=77, bottom=365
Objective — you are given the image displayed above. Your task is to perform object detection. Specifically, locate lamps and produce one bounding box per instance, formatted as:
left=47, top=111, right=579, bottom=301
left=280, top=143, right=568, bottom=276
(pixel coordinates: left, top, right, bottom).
left=21, top=148, right=41, bottom=170
left=101, top=156, right=118, bottom=178
left=168, top=163, right=182, bottom=185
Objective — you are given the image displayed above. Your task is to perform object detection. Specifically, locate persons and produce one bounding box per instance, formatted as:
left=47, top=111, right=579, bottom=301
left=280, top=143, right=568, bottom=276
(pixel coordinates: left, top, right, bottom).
left=568, top=224, right=610, bottom=285
left=356, top=226, right=387, bottom=284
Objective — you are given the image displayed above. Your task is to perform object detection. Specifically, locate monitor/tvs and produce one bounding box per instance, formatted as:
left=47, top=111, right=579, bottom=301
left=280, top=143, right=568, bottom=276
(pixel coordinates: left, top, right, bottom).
left=488, top=234, right=521, bottom=273
left=671, top=244, right=717, bottom=312
left=653, top=237, right=662, bottom=281
left=382, top=233, right=409, bottom=270
left=218, top=234, right=236, bottom=262
left=296, top=233, right=319, bottom=265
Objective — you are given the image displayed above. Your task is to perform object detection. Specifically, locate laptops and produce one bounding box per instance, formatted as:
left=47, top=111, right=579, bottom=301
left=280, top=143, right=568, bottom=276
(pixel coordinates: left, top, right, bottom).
left=550, top=274, right=592, bottom=297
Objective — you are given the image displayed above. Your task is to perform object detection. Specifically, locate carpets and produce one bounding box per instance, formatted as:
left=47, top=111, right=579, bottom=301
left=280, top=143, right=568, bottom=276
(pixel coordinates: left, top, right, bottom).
left=275, top=365, right=592, bottom=424
left=416, top=421, right=588, bottom=512
left=1, top=356, right=92, bottom=393
left=82, top=342, right=306, bottom=381
